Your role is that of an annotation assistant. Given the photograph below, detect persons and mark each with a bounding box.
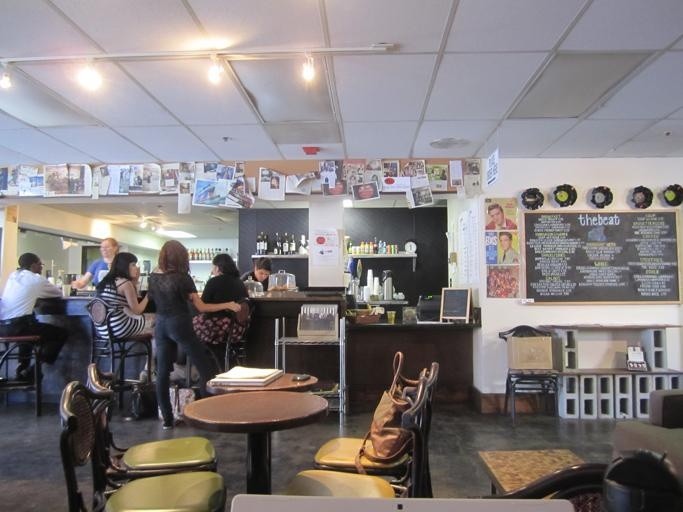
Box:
[93,253,156,380]
[429,169,436,180]
[487,270,518,298]
[240,258,273,291]
[71,238,122,290]
[486,204,517,230]
[440,169,447,180]
[149,241,241,430]
[499,232,519,264]
[170,253,250,381]
[0,253,68,379]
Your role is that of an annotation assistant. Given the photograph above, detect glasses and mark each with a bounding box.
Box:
[34,262,46,268]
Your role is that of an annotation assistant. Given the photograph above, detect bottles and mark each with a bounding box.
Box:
[346,236,398,254]
[255,230,307,255]
[382,270,393,300]
[186,247,229,260]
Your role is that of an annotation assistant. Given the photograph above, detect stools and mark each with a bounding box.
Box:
[647,389,682,428]
[0,335,43,417]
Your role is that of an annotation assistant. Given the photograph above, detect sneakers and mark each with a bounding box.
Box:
[163,421,180,430]
[139,362,199,384]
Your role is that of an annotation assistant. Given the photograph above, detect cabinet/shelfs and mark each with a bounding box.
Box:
[273,317,350,426]
[344,254,417,305]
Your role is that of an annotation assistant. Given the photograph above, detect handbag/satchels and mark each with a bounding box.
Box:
[356,351,431,461]
[158,386,195,422]
[128,389,157,421]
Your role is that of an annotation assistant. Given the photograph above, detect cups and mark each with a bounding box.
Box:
[63,285,71,296]
[363,269,379,301]
[387,312,396,324]
[402,307,417,322]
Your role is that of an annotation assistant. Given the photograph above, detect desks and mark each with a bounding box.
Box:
[477,448,586,500]
[183,390,329,496]
[205,372,321,396]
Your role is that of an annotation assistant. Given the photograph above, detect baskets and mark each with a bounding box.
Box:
[345,307,382,324]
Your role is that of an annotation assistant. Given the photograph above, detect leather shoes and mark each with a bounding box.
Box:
[19,368,44,381]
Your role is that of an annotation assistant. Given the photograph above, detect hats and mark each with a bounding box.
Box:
[18,253,38,270]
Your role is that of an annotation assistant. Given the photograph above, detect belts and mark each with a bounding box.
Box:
[1,313,36,326]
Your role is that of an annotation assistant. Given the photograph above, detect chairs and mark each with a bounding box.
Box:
[84,297,153,412]
[185,296,255,390]
[467,462,610,511]
[283,375,430,498]
[312,361,440,498]
[498,325,561,429]
[85,362,218,490]
[57,380,227,512]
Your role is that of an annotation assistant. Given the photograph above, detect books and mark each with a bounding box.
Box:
[210,362,283,388]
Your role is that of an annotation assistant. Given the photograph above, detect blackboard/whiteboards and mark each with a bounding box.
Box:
[440,287,471,320]
[520,207,683,305]
